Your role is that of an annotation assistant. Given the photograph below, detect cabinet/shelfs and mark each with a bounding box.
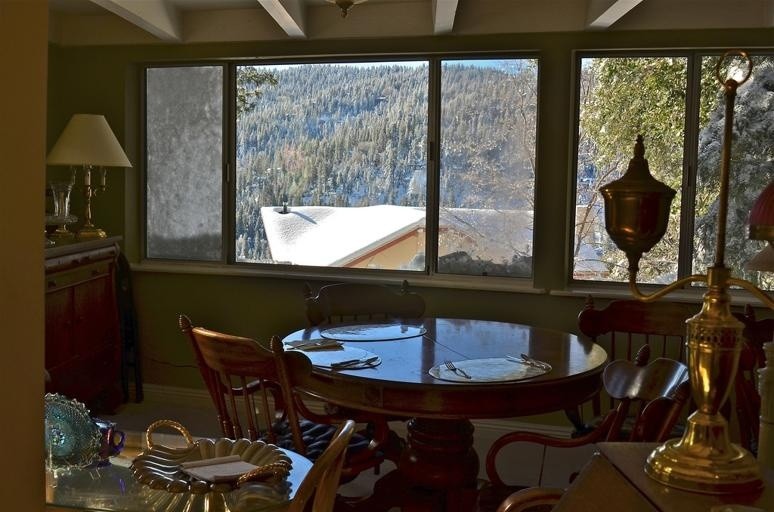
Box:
[45,259,120,407]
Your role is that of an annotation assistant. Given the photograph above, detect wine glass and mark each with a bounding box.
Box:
[50,181,79,237]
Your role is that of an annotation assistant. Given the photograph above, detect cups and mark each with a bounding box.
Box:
[94,418,126,469]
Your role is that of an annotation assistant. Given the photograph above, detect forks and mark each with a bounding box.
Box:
[442,358,475,380]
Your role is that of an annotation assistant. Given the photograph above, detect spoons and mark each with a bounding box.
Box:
[506,351,551,373]
[331,354,380,369]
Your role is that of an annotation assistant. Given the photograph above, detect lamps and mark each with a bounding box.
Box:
[598,46,772,497]
[45,110,134,239]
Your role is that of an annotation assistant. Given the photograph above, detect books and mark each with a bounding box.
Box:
[179,455,263,483]
